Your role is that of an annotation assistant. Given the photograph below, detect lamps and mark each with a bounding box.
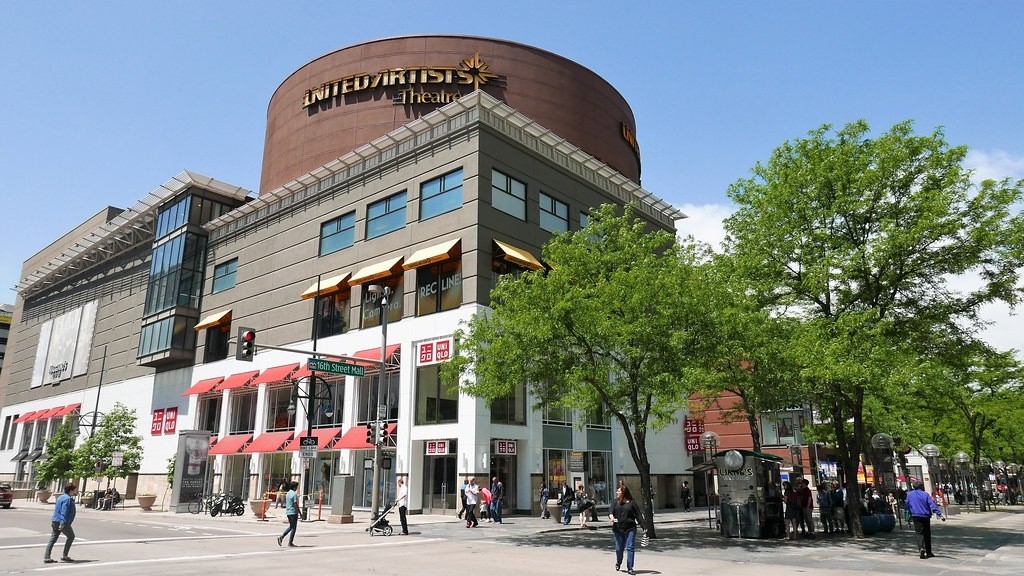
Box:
[77,244,78,247]
[49,263,51,266]
[26,279,28,281]
[109,222,112,225]
[130,210,132,212]
[92,234,94,236]
[62,254,64,256]
[15,286,17,288]
[37,271,39,274]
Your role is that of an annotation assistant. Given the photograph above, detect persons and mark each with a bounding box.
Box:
[457,476,504,528]
[538,482,551,519]
[767,473,845,541]
[277,481,303,547]
[905,480,946,559]
[617,479,625,489]
[857,496,894,517]
[44,482,75,563]
[607,484,648,575]
[274,477,289,510]
[397,478,408,535]
[680,481,691,513]
[557,479,572,524]
[931,482,948,520]
[1017,492,1023,504]
[650,483,656,516]
[95,487,121,511]
[576,484,589,528]
[998,490,1005,500]
[954,489,978,506]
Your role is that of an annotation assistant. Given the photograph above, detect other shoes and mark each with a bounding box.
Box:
[399,533,408,535]
[616,564,620,570]
[97,507,102,510]
[288,542,297,547]
[101,508,107,510]
[564,522,568,525]
[783,536,790,540]
[458,514,461,519]
[277,537,282,545]
[547,516,549,518]
[466,521,472,528]
[920,549,926,559]
[465,515,467,520]
[582,524,587,528]
[44,558,53,563]
[496,521,502,524]
[628,570,636,575]
[473,522,478,527]
[61,556,74,562]
[542,516,545,519]
[926,552,934,558]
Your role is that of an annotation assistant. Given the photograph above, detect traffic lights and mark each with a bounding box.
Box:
[363,458,374,470]
[381,458,392,470]
[236,325,255,362]
[364,424,376,445]
[378,422,389,444]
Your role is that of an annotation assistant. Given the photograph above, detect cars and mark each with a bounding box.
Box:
[0,485,13,508]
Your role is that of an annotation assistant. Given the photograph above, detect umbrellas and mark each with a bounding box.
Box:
[896,474,923,483]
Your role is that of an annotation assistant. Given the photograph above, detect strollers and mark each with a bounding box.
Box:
[366,500,398,536]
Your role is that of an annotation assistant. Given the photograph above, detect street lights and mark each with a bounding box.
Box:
[955,451,972,514]
[287,274,336,525]
[699,431,721,532]
[367,283,394,526]
[920,444,940,507]
[70,344,107,505]
[871,433,894,501]
[995,460,1006,506]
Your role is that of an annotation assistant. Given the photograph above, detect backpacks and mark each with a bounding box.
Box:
[789,491,800,509]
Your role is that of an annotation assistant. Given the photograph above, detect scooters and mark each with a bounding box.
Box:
[210,493,245,518]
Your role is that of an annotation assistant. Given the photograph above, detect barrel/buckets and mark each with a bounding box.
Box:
[873,514,895,531]
[860,515,880,534]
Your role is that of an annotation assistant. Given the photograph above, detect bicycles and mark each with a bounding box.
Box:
[187,492,220,514]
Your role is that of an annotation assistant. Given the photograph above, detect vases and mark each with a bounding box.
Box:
[55,493,63,500]
[873,514,896,533]
[861,515,881,535]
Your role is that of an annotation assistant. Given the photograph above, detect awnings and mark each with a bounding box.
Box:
[11,451,53,462]
[209,424,396,455]
[493,238,544,270]
[13,404,80,423]
[194,309,232,330]
[300,237,461,300]
[181,344,399,396]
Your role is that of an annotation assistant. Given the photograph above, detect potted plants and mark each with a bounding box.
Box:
[137,493,157,510]
[37,489,53,503]
[80,494,94,508]
[249,496,273,517]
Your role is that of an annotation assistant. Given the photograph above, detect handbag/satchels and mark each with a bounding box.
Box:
[641,533,649,547]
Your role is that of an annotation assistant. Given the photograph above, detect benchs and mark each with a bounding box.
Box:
[104,493,127,510]
